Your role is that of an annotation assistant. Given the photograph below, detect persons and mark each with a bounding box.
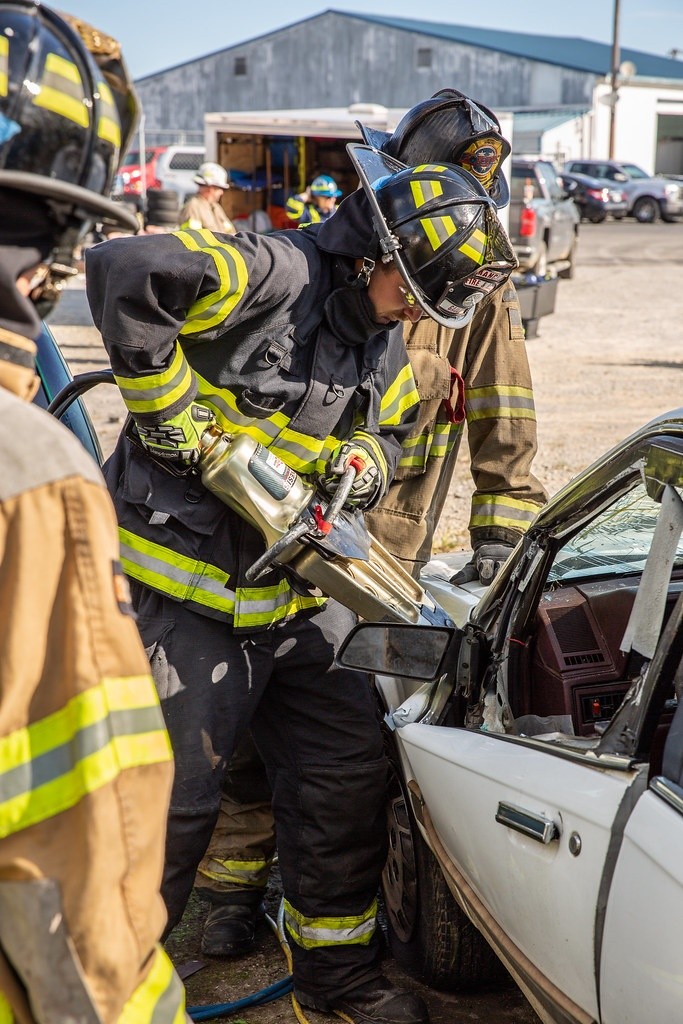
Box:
[284,174,343,230]
[83,161,518,1023]
[0,0,204,1024]
[188,86,552,961]
[178,161,238,238]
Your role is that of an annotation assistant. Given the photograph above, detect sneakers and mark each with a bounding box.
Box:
[200,902,257,956]
[292,978,430,1024]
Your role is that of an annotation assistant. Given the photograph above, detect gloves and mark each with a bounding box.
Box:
[135,403,216,475]
[317,441,381,508]
[449,537,516,585]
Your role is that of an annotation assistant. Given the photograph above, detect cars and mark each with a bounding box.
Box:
[109,146,207,221]
[554,171,628,227]
[333,406,683,1024]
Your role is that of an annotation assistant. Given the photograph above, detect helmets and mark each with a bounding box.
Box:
[1,0,143,262]
[347,140,520,329]
[355,88,510,210]
[310,175,342,198]
[194,161,229,189]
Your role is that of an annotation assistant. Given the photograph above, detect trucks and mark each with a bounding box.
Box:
[200,103,512,322]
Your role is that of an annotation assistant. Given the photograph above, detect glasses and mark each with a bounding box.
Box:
[387,264,425,313]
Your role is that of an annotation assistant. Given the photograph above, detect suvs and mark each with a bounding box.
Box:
[566,159,683,225]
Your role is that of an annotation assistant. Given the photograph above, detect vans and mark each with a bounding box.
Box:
[509,157,586,282]
